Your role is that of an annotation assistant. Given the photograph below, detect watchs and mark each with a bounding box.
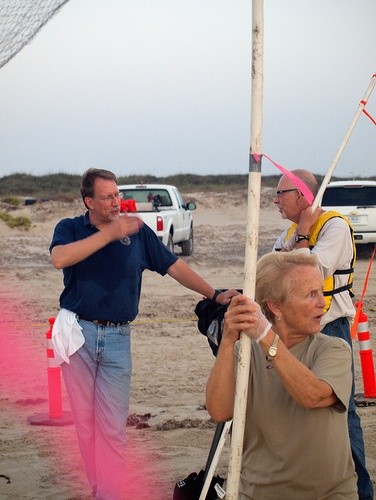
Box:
[294,233,310,243]
[266,333,279,360]
[212,289,221,302]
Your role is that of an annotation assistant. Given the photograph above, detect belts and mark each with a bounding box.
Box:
[91,318,131,328]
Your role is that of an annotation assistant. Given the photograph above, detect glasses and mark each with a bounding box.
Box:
[276,189,303,198]
[90,191,123,203]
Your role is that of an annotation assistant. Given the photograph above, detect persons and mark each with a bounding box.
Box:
[206,252,359,500]
[49,167,242,500]
[272,169,374,500]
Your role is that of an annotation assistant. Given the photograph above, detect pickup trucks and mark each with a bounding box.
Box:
[116,183,196,257]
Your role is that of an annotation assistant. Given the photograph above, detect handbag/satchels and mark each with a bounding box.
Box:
[174,468,224,500]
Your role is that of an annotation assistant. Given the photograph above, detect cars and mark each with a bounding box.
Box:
[318,180,376,244]
